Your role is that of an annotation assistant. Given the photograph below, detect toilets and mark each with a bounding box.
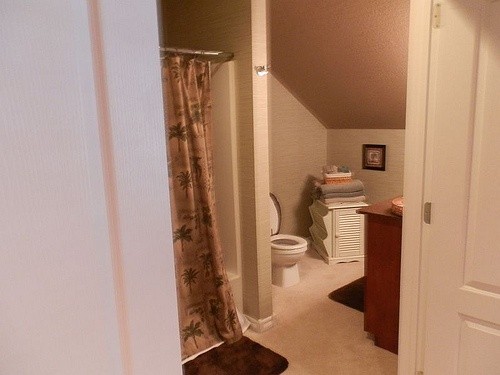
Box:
[270,193,308,288]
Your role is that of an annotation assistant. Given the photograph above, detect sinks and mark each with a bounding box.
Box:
[392,196,404,216]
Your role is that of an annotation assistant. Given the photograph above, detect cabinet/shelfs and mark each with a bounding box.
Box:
[356,193,404,355]
[309,199,370,265]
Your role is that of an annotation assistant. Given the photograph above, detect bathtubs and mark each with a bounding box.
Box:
[226,271,243,314]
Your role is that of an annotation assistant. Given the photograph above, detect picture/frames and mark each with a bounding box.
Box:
[362,144,386,171]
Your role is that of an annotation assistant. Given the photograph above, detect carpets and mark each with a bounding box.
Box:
[182,336,289,375]
[328,276,364,312]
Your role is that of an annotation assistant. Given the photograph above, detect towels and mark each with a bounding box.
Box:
[311,180,367,205]
